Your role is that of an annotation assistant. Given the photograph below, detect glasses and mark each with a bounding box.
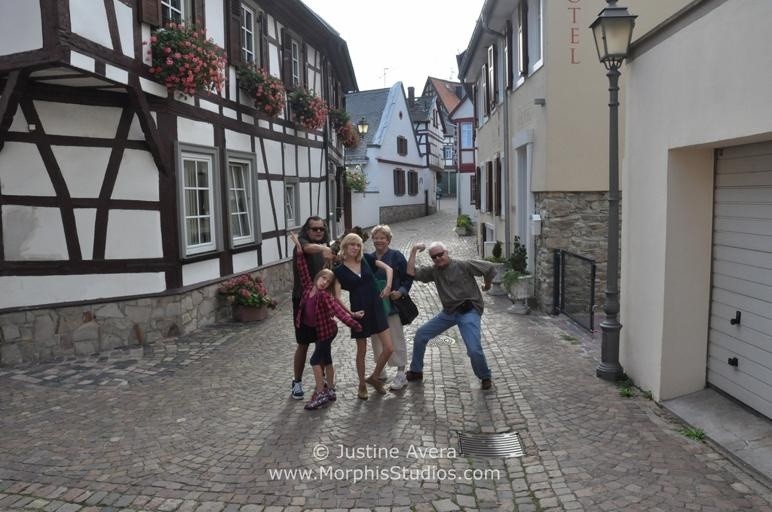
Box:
[431,252,444,259]
[310,227,325,231]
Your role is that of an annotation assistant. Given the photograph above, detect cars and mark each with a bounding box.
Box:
[436,186,443,199]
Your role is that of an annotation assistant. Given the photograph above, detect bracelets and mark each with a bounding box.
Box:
[352,312,355,317]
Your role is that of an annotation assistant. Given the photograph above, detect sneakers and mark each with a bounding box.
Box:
[482,379,491,389]
[290,374,337,409]
[358,369,423,399]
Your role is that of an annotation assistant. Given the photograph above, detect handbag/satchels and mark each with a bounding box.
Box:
[393,294,418,324]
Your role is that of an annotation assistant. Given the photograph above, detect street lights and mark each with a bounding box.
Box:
[590,0,638,381]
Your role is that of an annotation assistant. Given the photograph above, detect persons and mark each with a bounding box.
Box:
[368,223,415,391]
[287,230,364,411]
[333,233,395,400]
[289,215,336,401]
[404,241,498,390]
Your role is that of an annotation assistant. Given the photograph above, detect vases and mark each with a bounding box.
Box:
[231,303,268,322]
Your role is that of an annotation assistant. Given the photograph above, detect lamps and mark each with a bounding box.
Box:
[357,116,369,139]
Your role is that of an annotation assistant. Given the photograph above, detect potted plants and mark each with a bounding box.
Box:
[452,214,473,236]
[480,241,508,296]
[500,234,536,315]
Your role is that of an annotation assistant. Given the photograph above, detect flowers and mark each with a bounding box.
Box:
[217,272,278,311]
[142,13,359,149]
